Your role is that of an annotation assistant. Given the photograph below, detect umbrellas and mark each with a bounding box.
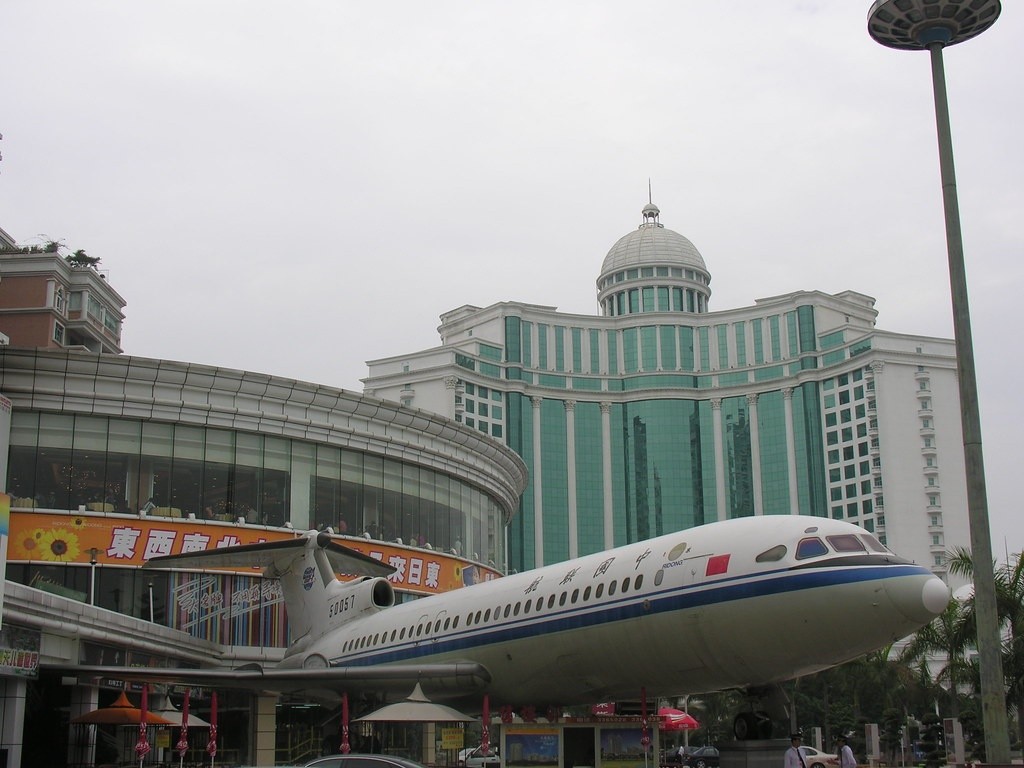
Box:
[640,689,650,768]
[482,696,489,768]
[659,708,698,762]
[207,692,218,768]
[70,690,174,724]
[349,681,479,721]
[177,687,190,768]
[136,684,150,768]
[150,696,213,727]
[340,690,350,755]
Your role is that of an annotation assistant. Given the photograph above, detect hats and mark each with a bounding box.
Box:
[788,733,802,739]
[837,734,849,741]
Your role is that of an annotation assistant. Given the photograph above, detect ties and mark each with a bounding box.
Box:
[840,749,842,768]
[797,748,806,768]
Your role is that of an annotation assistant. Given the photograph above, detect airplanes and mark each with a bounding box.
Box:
[0,513,952,741]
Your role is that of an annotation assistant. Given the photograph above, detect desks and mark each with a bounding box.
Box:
[214,514,235,521]
[86,502,114,512]
[13,498,38,508]
[150,507,181,518]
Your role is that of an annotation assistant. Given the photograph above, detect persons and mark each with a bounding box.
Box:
[677,745,685,762]
[784,736,807,768]
[144,498,156,515]
[206,505,215,520]
[829,736,857,768]
[336,515,347,534]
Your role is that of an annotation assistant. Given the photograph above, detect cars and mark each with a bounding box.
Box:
[301,753,427,768]
[799,746,840,768]
[459,748,477,767]
[465,743,500,768]
[661,747,700,763]
[683,746,720,768]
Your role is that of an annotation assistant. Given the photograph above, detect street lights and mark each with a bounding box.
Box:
[867,0,1016,768]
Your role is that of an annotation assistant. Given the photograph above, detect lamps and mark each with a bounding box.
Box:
[78,506,518,574]
